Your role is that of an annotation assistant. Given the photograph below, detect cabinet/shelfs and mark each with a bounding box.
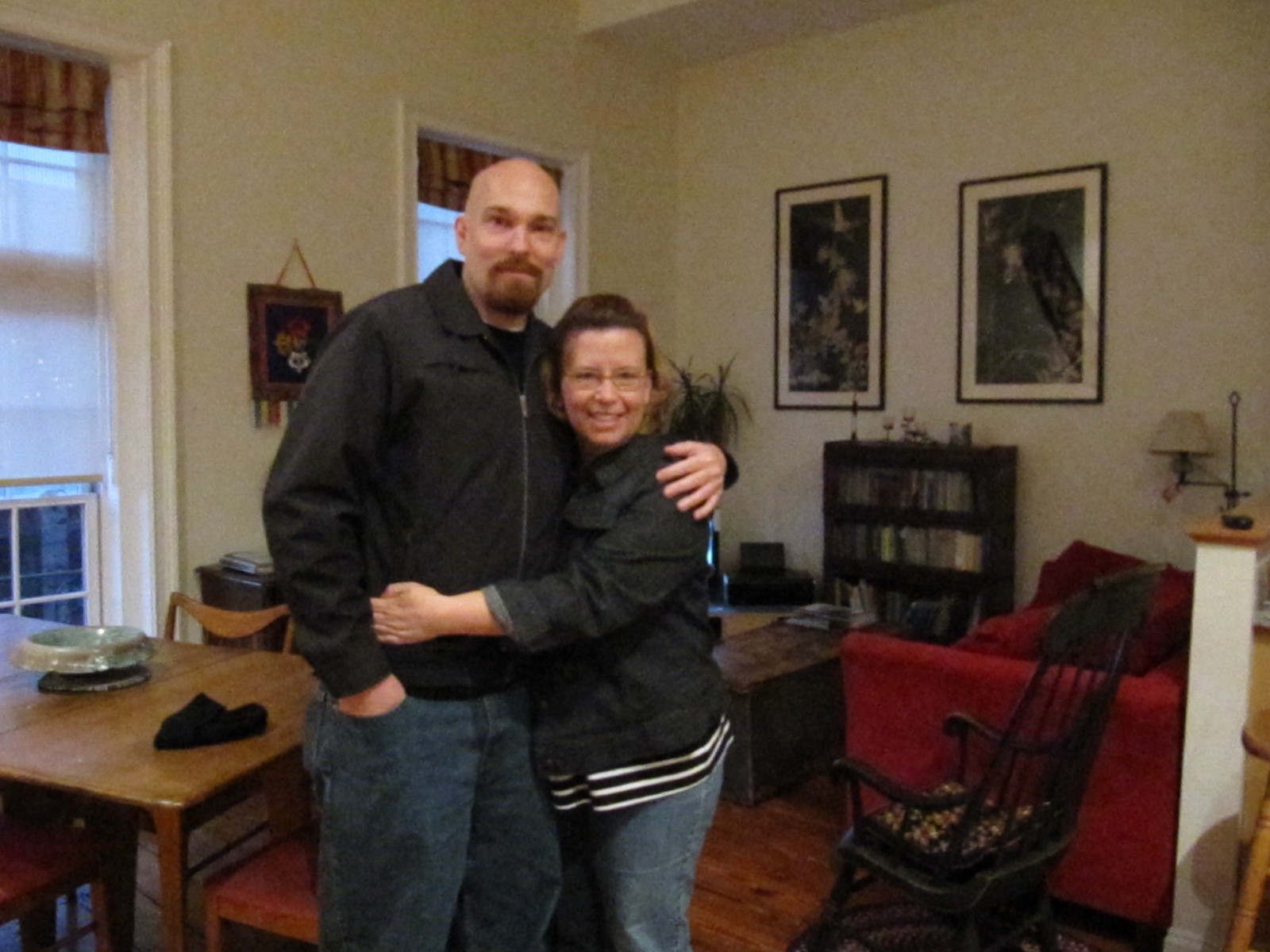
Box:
[824,440,1024,632]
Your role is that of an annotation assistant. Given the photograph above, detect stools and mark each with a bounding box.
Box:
[1225,711,1270,952]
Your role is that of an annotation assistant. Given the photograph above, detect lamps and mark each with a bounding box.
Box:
[1151,413,1247,514]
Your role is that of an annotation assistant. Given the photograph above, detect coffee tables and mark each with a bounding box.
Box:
[711,620,897,805]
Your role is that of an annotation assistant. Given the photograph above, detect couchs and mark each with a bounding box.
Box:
[849,542,1202,926]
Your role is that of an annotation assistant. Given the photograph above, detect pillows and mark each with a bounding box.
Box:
[952,601,1057,654]
[1075,573,1195,671]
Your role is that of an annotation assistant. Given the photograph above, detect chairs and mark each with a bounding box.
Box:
[821,563,1170,952]
[0,590,319,952]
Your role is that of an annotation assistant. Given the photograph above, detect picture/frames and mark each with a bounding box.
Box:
[247,284,348,404]
[954,162,1108,406]
[770,173,886,411]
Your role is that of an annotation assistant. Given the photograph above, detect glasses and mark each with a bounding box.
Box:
[560,367,654,392]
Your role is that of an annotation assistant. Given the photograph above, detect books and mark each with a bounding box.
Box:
[834,467,984,641]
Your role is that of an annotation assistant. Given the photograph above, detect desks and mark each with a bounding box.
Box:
[0,613,322,952]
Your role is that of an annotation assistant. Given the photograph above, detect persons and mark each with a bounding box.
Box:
[371,294,734,952]
[259,155,741,951]
